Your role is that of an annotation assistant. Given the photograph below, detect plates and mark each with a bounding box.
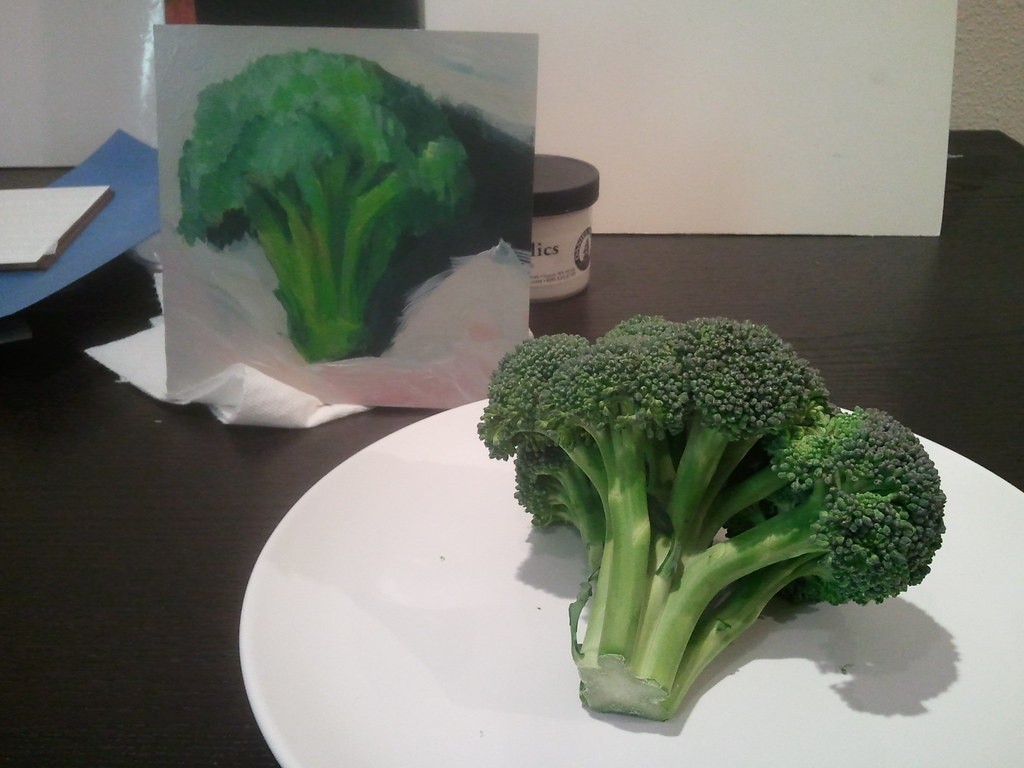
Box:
[236,397,1024,768]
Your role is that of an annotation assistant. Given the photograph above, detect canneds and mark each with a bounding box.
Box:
[531,155,599,302]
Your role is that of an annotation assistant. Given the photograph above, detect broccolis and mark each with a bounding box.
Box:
[476,315,947,720]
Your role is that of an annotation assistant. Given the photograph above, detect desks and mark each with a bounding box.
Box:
[0,128,1024,768]
